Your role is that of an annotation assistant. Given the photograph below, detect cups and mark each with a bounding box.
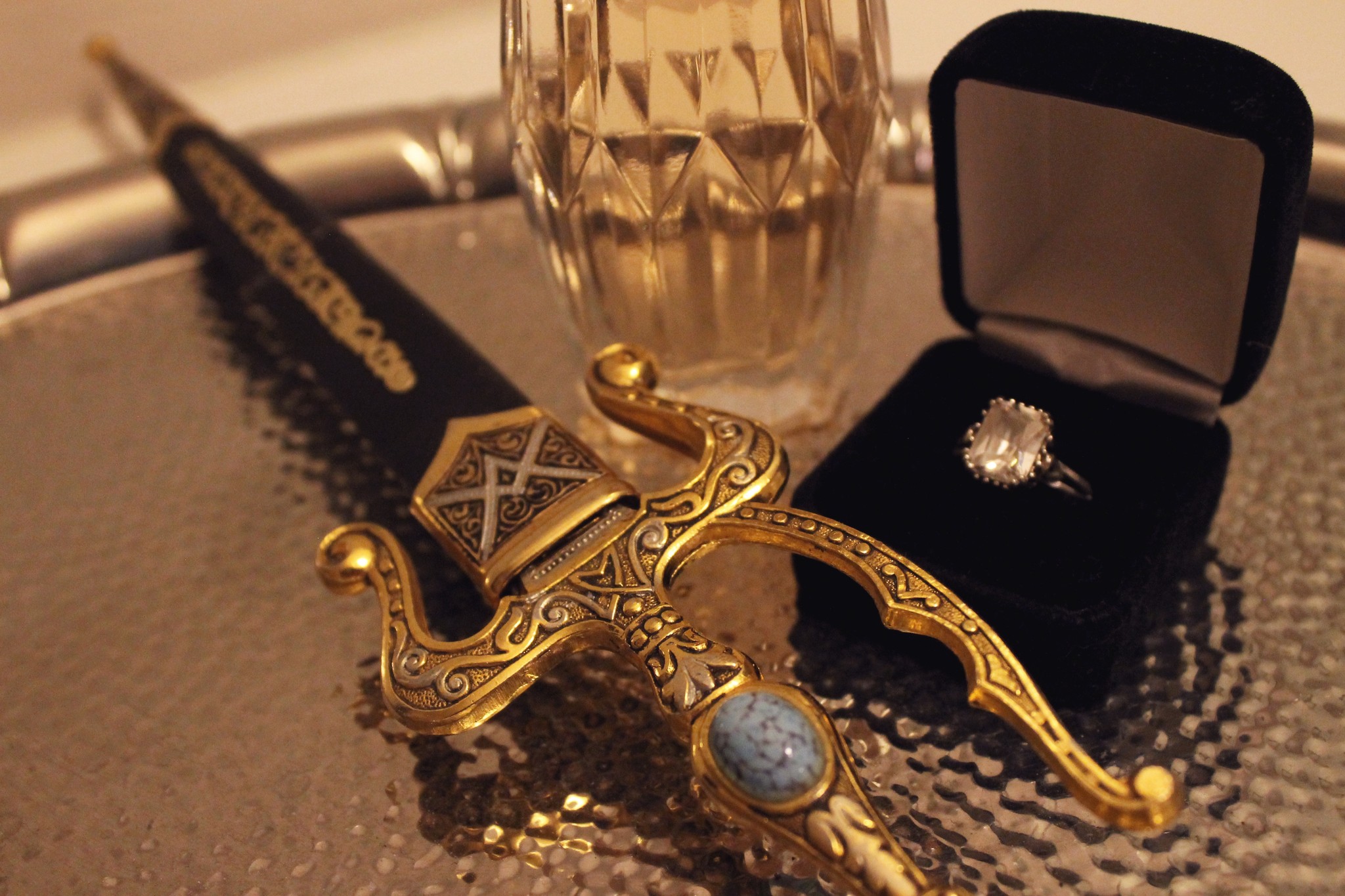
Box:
[500,0,896,442]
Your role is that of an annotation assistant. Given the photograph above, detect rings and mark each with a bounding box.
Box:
[961,398,1094,506]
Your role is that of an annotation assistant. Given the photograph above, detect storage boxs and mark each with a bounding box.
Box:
[784,11,1315,706]
